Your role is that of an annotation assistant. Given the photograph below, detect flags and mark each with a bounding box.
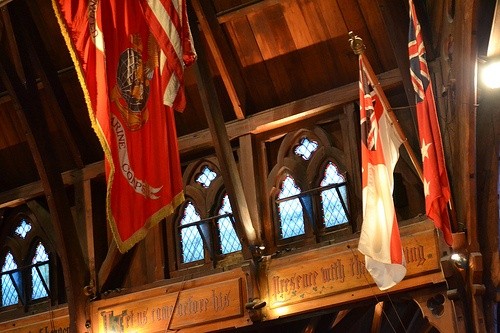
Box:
[139,0,199,115]
[408,5,458,246]
[355,53,405,293]
[54,1,186,253]
